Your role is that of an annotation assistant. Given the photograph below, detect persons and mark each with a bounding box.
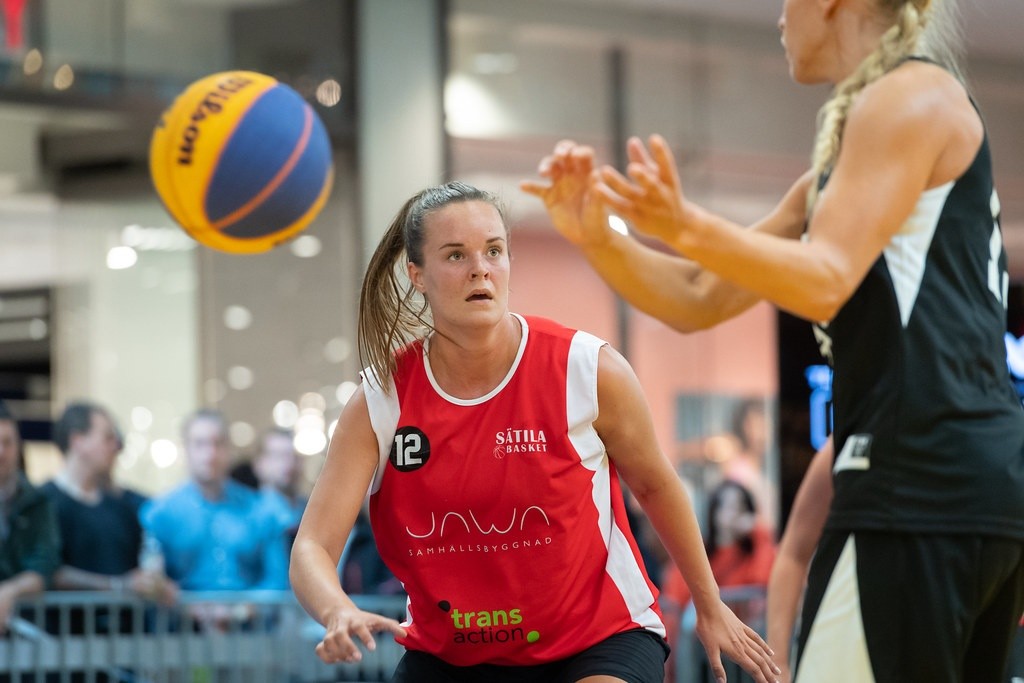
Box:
[516,0,1024,683]
[0,399,835,683]
[287,182,781,683]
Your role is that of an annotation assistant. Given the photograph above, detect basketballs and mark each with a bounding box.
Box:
[144,66,339,258]
